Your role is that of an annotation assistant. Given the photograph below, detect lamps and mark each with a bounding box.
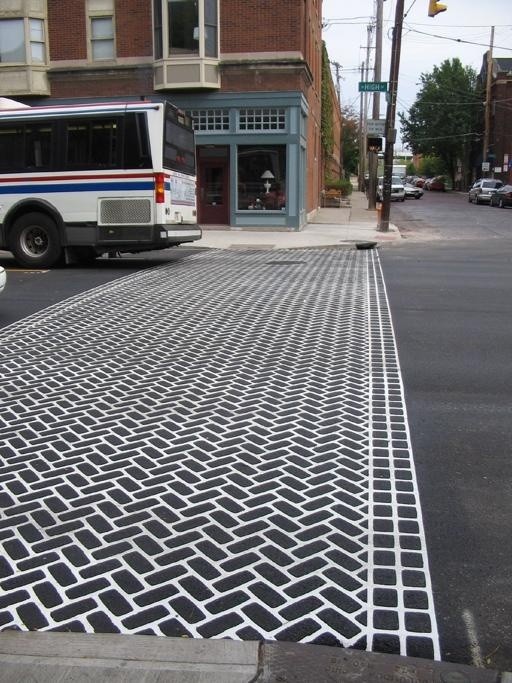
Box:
[260,169,275,193]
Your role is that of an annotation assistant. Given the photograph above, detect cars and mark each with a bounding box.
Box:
[364,172,453,201]
[489,183,512,208]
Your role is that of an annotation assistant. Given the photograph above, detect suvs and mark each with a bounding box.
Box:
[468,176,505,205]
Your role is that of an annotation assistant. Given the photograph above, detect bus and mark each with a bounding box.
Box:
[1,96,205,269]
